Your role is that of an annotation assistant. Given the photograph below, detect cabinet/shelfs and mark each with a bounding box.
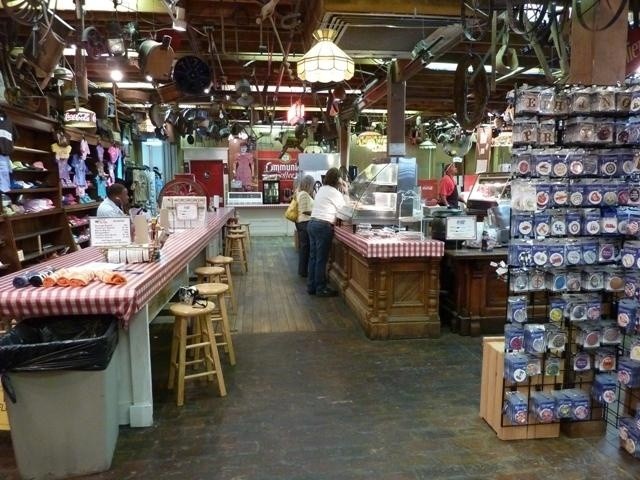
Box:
[0,100,130,336]
[336,156,416,225]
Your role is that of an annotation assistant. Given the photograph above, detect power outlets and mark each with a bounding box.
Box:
[159,0,188,32]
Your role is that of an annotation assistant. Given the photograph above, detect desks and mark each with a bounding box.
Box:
[326,224,617,341]
[0,204,237,429]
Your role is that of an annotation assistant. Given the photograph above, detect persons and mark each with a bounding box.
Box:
[292,174,315,278]
[435,162,468,210]
[96,182,139,238]
[232,141,256,192]
[306,167,349,298]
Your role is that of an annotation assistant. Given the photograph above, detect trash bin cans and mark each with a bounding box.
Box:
[0,315,118,480]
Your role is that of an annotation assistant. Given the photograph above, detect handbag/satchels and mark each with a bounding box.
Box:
[284,198,298,221]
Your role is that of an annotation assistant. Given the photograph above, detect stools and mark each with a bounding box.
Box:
[166,216,252,407]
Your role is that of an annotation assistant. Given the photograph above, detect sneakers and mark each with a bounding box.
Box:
[307,286,338,297]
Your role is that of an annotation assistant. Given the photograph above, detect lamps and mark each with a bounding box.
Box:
[419,137,437,150]
[356,131,383,148]
[296,11,355,85]
